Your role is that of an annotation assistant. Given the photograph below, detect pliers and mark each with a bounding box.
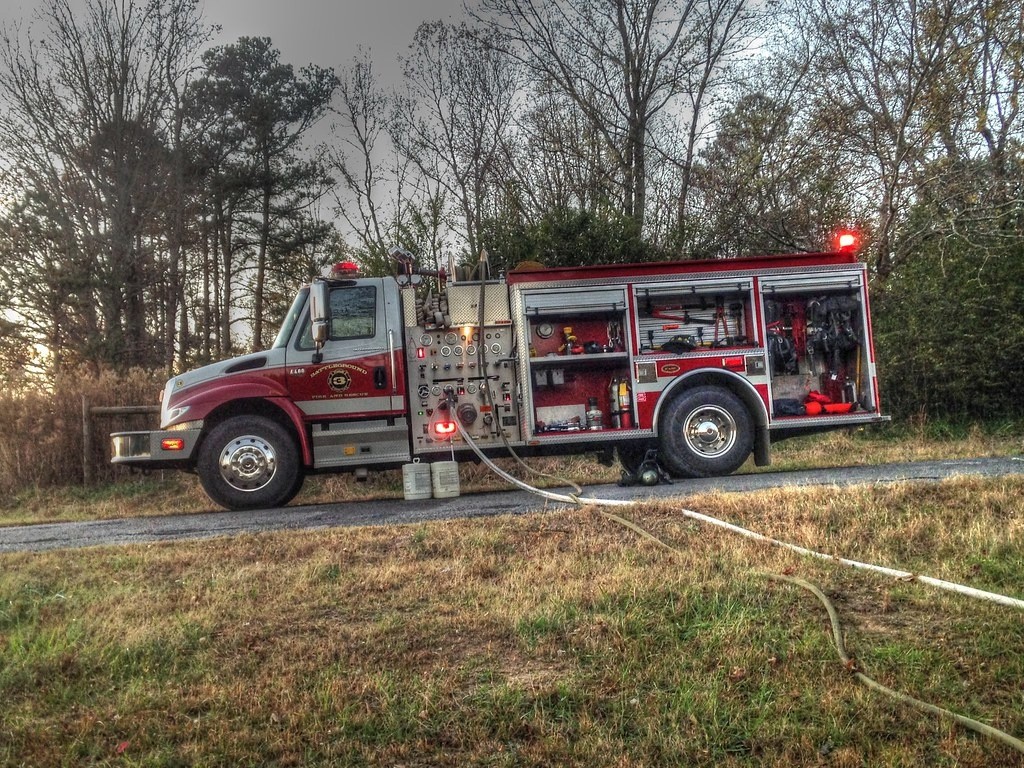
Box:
[636,303,716,324]
[714,307,729,346]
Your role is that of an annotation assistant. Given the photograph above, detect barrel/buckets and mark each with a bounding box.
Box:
[401,462,431,500]
[430,460,460,499]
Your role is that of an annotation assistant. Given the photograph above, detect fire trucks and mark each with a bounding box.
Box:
[110,226,893,512]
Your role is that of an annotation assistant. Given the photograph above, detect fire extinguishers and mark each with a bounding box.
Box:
[608,375,620,429]
[618,376,633,429]
